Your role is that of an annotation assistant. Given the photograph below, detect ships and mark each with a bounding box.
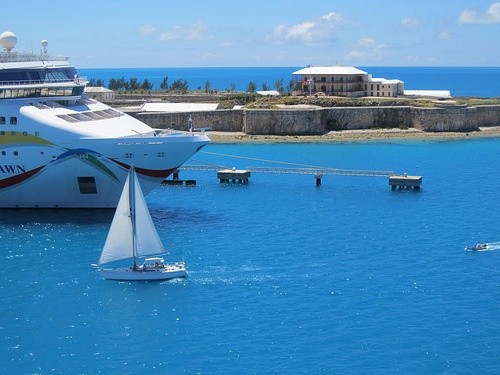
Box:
[1,32,211,211]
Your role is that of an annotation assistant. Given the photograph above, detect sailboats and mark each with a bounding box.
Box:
[89,162,190,284]
